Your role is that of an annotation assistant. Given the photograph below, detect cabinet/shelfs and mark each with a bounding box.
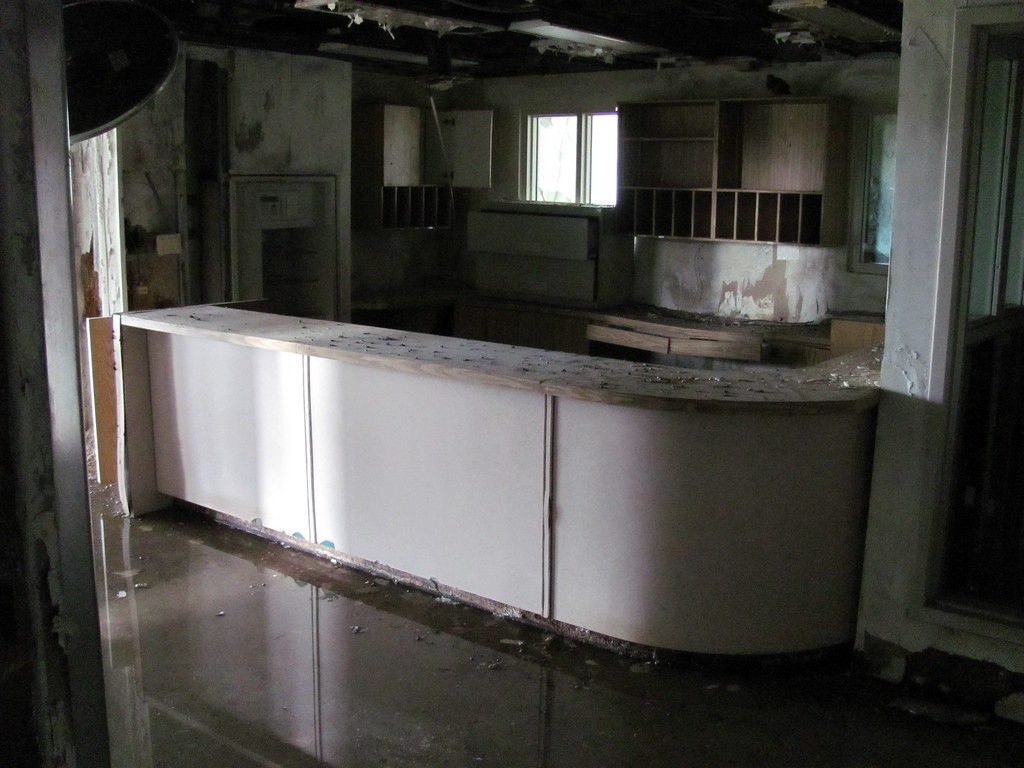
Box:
[368,100,496,232]
[610,94,852,249]
